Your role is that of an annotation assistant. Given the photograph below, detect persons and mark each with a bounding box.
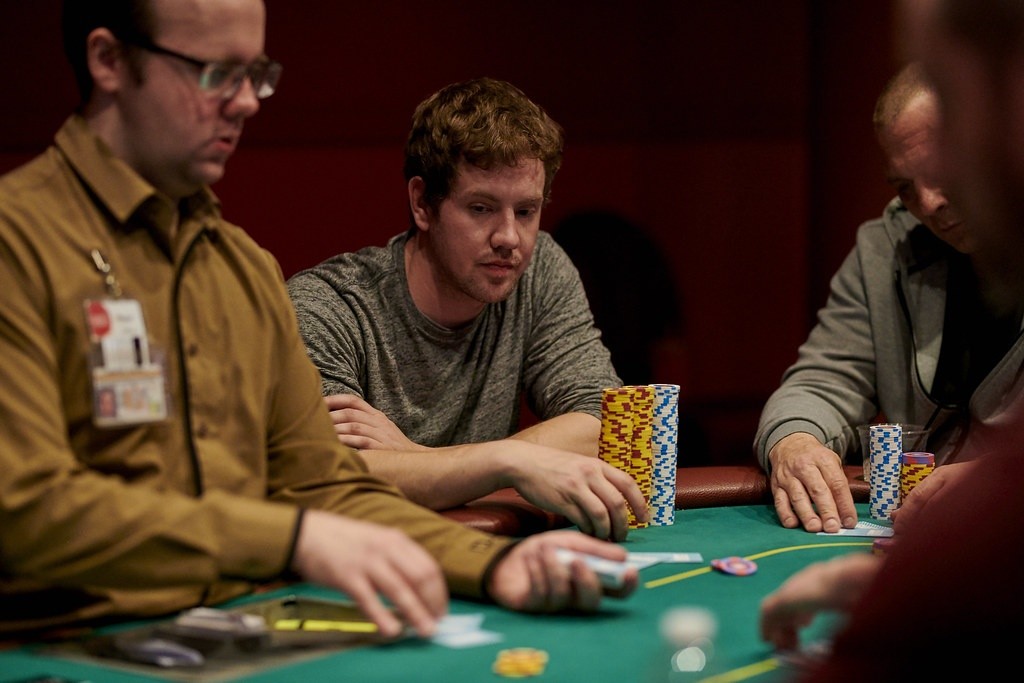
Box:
[752,57,1024,534]
[288,74,649,543]
[756,2,1023,682]
[0,0,640,641]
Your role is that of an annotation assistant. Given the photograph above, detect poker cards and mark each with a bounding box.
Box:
[816,520,895,538]
[554,548,704,591]
[403,612,505,651]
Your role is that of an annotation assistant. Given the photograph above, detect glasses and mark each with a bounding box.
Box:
[118,33,281,99]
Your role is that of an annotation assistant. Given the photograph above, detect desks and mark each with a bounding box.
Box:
[1,466,937,683]
